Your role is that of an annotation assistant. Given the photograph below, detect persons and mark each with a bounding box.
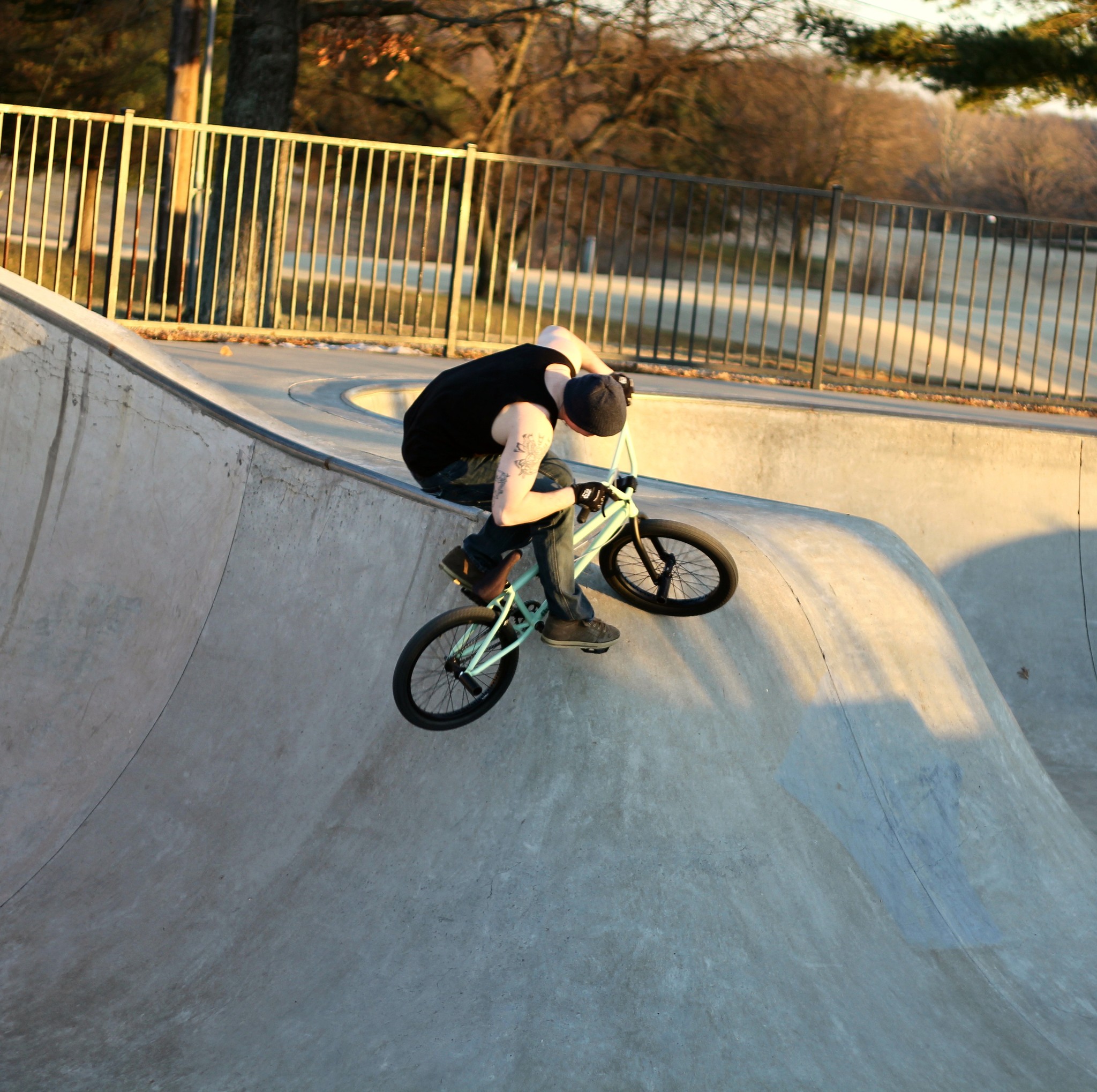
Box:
[401,325,633,648]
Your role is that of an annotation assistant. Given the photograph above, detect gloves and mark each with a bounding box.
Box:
[610,373,634,407]
[572,482,609,512]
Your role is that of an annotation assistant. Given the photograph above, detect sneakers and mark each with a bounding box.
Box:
[439,546,508,590]
[541,616,621,649]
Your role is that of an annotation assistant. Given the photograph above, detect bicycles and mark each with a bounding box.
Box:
[391,405,740,732]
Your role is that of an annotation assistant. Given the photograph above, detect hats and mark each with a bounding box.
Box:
[563,374,627,437]
